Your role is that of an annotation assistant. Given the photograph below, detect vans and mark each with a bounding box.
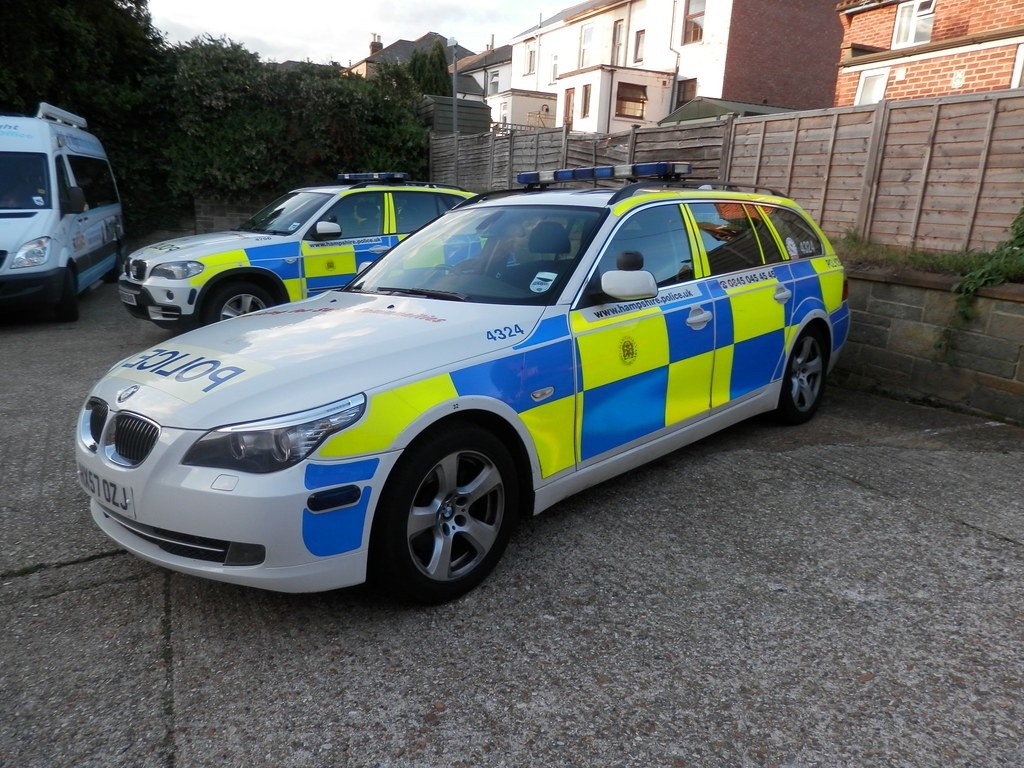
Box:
[0,100,126,324]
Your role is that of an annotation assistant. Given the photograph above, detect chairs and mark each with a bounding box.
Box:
[515,220,572,299]
[357,202,380,229]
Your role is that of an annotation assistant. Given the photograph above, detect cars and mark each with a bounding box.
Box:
[115,171,532,334]
[70,158,851,611]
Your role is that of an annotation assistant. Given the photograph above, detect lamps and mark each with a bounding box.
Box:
[542,104,549,112]
